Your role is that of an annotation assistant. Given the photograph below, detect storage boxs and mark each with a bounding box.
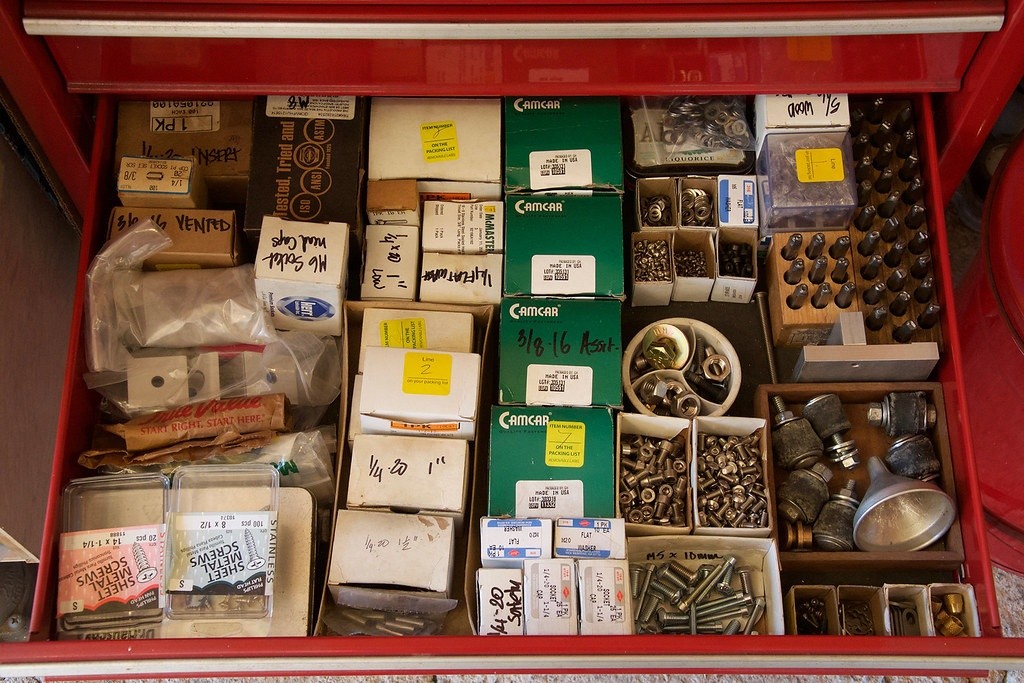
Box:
[62,93,981,637]
